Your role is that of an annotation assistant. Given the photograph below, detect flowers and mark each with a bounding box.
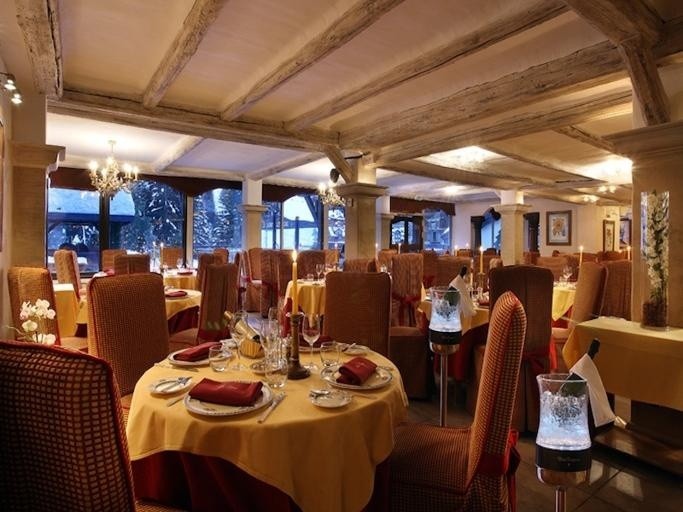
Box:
[643,189,669,330]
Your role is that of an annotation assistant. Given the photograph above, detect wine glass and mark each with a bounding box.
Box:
[176,257,183,272]
[302,311,320,374]
[231,312,250,372]
[316,263,324,283]
[259,319,282,382]
[268,307,283,327]
[563,266,570,287]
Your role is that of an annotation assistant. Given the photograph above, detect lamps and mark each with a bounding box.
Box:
[88,140,140,201]
[0,72,23,107]
[318,178,348,207]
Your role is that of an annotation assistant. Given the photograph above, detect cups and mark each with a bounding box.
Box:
[264,359,289,388]
[207,346,232,371]
[306,274,314,281]
[320,341,341,366]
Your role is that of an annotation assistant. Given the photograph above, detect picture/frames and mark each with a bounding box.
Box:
[619,218,631,249]
[546,209,572,245]
[603,220,616,253]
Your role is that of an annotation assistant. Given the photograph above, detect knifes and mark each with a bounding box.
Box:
[259,391,286,423]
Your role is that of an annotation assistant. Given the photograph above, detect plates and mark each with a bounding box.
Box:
[310,389,351,409]
[184,378,274,417]
[320,363,390,389]
[169,343,230,368]
[151,375,192,396]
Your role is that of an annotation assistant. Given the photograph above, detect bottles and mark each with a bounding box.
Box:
[223,309,261,342]
[434,267,469,320]
[553,340,600,428]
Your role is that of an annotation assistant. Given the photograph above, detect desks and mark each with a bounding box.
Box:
[562,315,682,485]
